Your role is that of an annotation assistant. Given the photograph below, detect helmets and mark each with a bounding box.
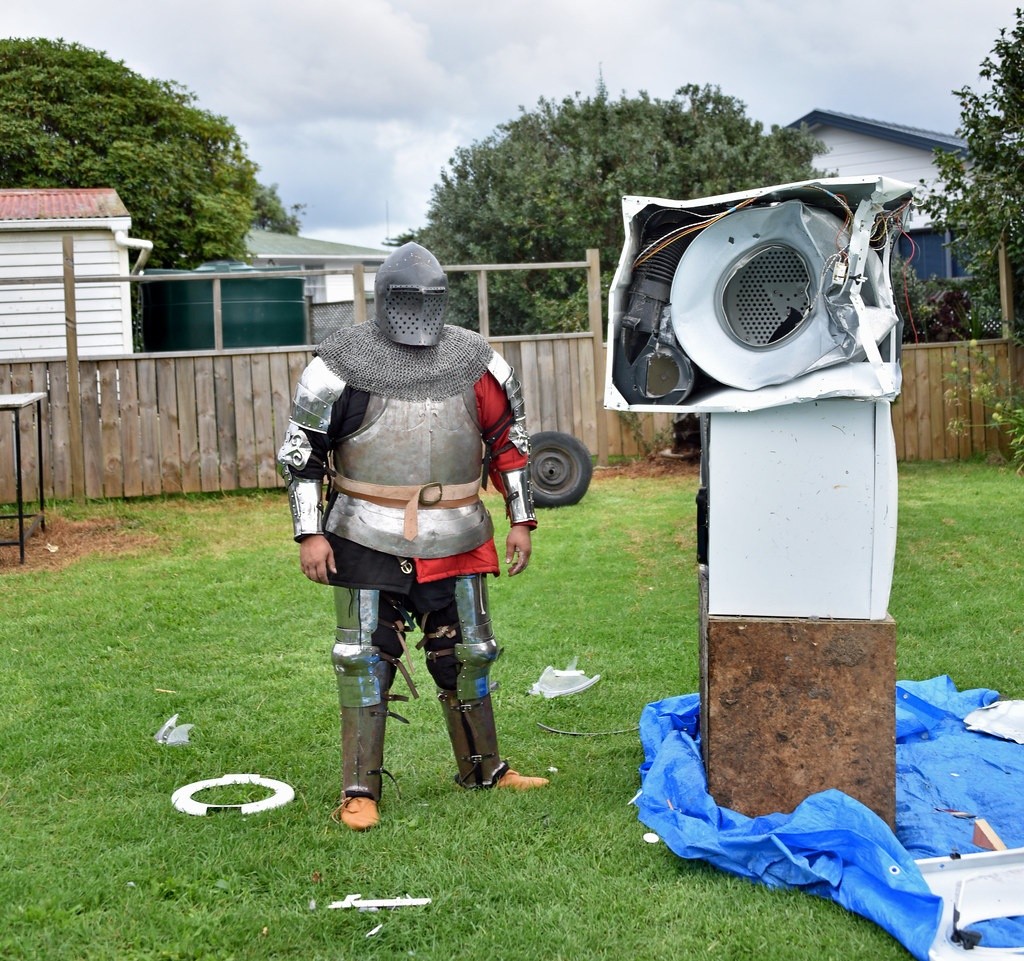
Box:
[374,242,449,345]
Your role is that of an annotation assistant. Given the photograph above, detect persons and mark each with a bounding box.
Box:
[275,239,550,829]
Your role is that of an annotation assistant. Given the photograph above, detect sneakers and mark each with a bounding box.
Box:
[332,797,380,831]
[495,768,549,790]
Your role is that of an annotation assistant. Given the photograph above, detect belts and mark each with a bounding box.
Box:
[331,467,481,542]
